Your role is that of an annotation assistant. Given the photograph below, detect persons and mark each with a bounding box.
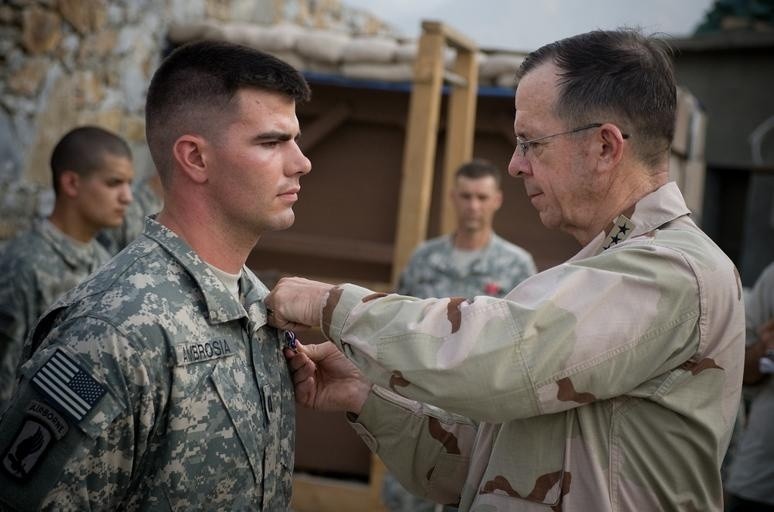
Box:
[264,29,744,512]
[382,161,536,512]
[0,126,139,400]
[0,37,316,512]
[724,261,774,512]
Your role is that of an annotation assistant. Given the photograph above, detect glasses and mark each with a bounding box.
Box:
[517,123,629,158]
